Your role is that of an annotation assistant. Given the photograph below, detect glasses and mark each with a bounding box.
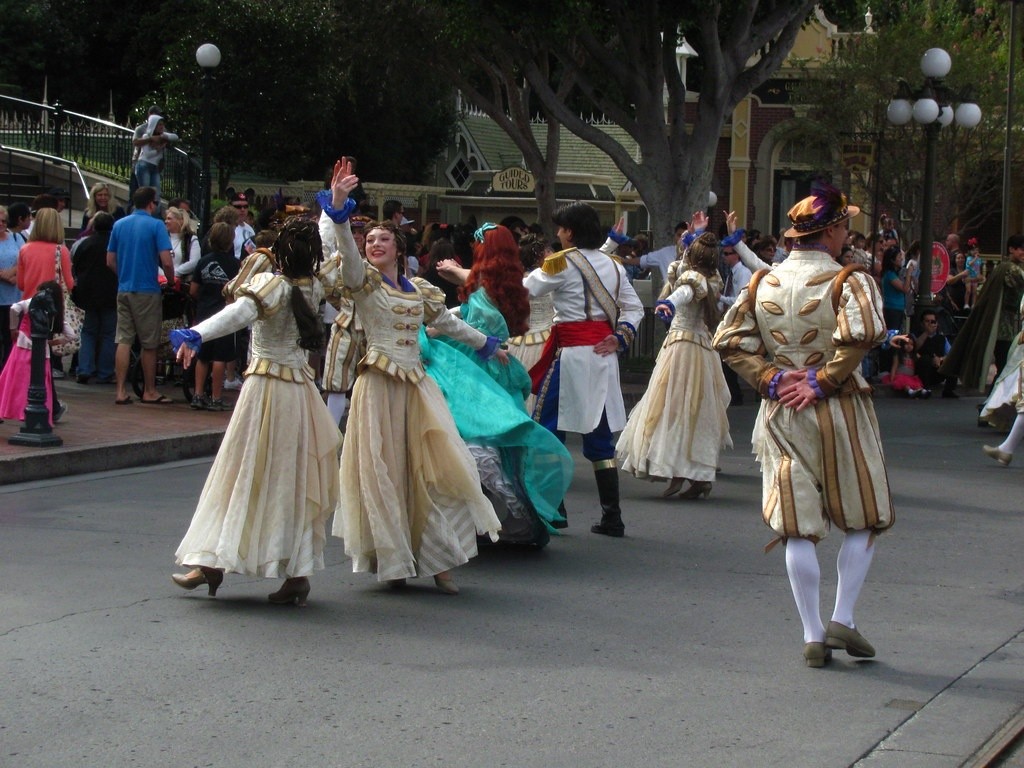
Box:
[397,212,405,215]
[232,205,248,209]
[723,252,737,256]
[524,230,528,235]
[764,248,777,253]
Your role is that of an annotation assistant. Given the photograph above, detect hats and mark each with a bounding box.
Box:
[230,192,247,201]
[350,216,373,227]
[48,188,70,200]
[783,179,860,237]
[399,216,415,225]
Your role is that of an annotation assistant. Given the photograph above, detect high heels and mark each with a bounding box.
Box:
[664,476,684,496]
[269,576,311,607]
[682,481,712,500]
[172,566,223,597]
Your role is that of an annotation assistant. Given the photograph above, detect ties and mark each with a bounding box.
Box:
[723,269,733,311]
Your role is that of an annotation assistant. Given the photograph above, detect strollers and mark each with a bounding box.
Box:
[130,281,211,399]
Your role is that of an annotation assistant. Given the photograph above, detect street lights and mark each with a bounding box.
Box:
[707,192,718,232]
[196,43,223,235]
[886,46,983,338]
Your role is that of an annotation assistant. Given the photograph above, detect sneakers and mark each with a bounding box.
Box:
[52,403,67,422]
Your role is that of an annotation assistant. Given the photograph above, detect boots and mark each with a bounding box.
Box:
[532,453,567,528]
[591,458,624,537]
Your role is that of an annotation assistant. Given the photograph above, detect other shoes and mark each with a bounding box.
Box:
[942,391,959,398]
[983,445,1013,467]
[52,366,243,410]
[910,389,921,398]
[922,390,931,399]
[804,641,831,668]
[434,572,460,593]
[964,304,970,312]
[825,621,876,657]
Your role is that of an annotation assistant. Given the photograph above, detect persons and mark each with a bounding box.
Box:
[134,116,179,214]
[168,217,341,606]
[127,105,172,214]
[0,157,1024,594]
[710,184,893,667]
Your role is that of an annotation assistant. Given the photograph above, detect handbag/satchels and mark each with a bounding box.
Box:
[48,245,85,356]
[159,314,188,361]
